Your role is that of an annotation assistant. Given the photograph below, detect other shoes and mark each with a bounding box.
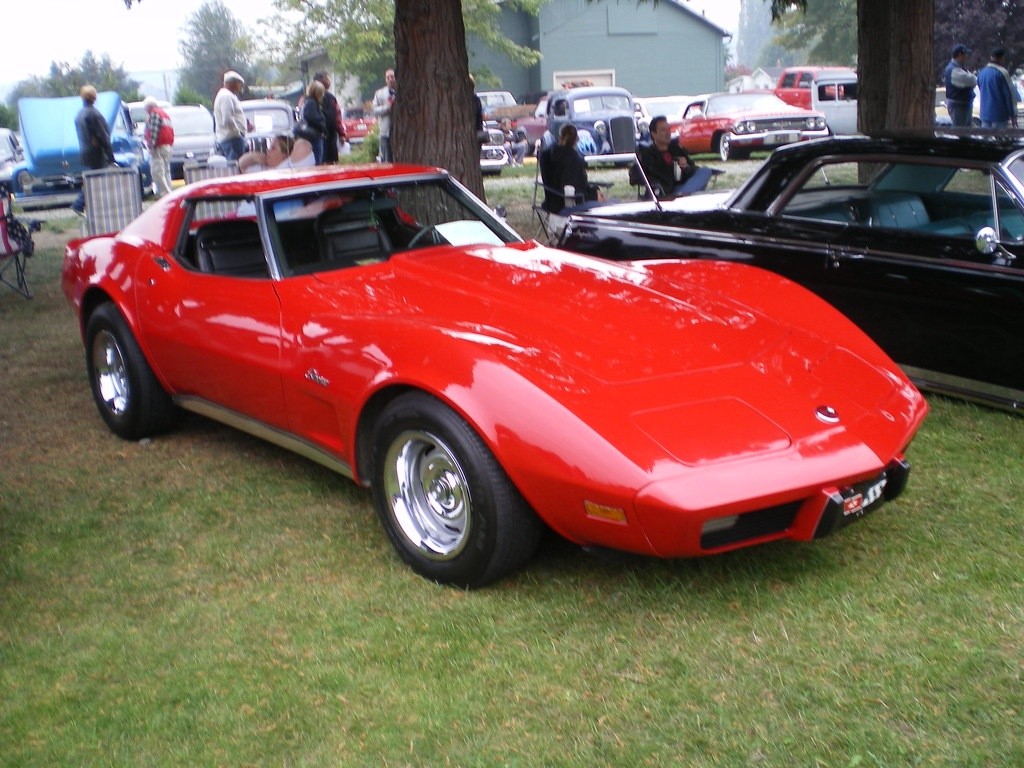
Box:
[71,206,87,218]
[516,161,524,168]
[510,161,515,169]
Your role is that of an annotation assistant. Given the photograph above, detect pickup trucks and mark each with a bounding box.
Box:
[742,67,852,109]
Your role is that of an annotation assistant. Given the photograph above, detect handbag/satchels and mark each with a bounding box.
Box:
[293,120,319,143]
[5,215,34,258]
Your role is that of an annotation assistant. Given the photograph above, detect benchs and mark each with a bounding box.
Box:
[803,190,929,229]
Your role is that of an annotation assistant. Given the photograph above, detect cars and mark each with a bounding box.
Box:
[478,91,519,172]
[10,93,152,211]
[667,89,831,160]
[555,139,1024,420]
[511,85,653,169]
[125,102,216,179]
[937,86,1024,135]
[0,129,23,195]
[809,74,858,137]
[342,108,376,143]
[238,98,299,154]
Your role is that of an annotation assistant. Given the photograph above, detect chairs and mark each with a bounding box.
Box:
[917,210,1024,241]
[184,159,242,186]
[81,167,144,238]
[0,183,40,299]
[629,162,726,203]
[534,180,614,247]
[193,218,268,281]
[315,207,396,275]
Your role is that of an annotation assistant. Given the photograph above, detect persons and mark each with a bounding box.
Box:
[975,47,1017,127]
[236,70,349,176]
[468,74,549,166]
[70,86,114,217]
[943,42,979,140]
[373,69,396,164]
[540,123,610,206]
[628,117,712,201]
[213,71,246,166]
[142,97,174,201]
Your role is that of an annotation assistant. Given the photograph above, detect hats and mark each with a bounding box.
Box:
[990,47,1006,58]
[951,44,972,58]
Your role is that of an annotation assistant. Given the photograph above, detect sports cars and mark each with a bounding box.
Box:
[60,163,933,588]
